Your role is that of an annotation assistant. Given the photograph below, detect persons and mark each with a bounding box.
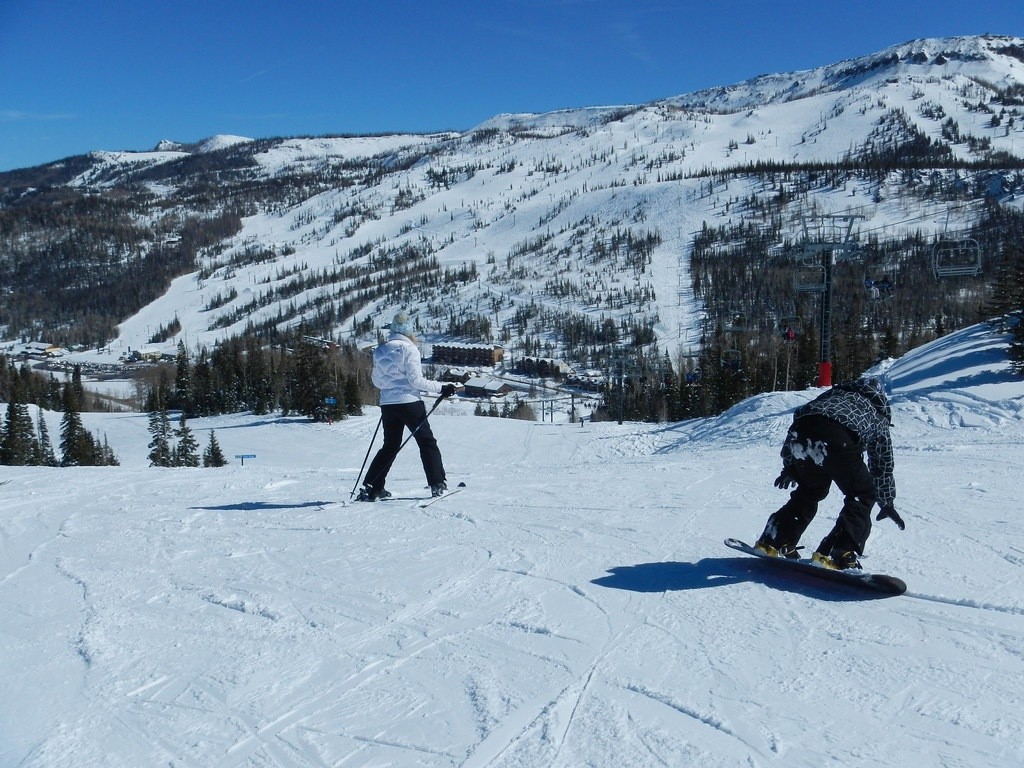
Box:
[360,312,456,501]
[567,406,576,415]
[579,417,584,428]
[583,402,596,409]
[754,378,905,571]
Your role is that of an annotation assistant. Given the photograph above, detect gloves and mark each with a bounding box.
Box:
[876,505,905,530]
[774,469,796,489]
[441,384,458,398]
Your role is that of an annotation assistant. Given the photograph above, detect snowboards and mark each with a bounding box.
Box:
[723,534,909,598]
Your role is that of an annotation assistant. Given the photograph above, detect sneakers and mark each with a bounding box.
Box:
[755,532,805,561]
[365,486,391,499]
[812,540,863,573]
[431,481,449,497]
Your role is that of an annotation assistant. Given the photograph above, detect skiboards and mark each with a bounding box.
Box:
[316,480,469,510]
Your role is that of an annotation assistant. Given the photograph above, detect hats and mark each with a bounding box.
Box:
[390,312,416,337]
